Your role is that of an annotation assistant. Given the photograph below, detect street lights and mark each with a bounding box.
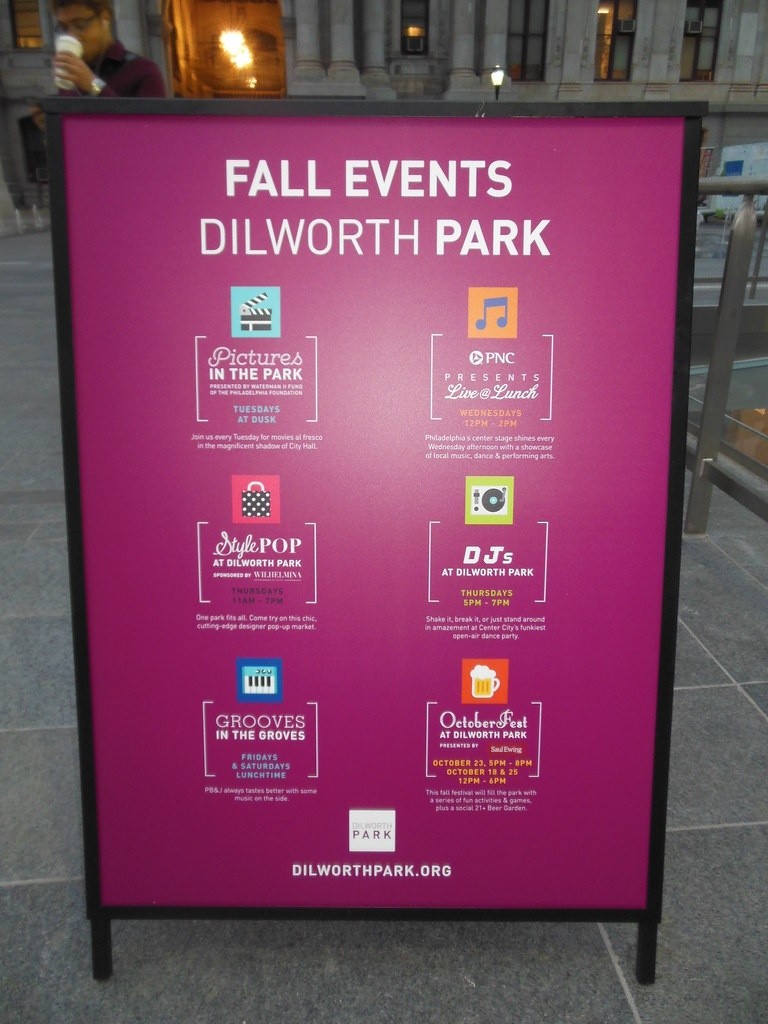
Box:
[490,63,504,100]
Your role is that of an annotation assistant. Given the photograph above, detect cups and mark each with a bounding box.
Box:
[54,36,84,90]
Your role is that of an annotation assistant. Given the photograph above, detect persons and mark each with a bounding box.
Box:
[29,0,165,133]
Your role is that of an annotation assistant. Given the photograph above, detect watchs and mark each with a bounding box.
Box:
[89,76,106,97]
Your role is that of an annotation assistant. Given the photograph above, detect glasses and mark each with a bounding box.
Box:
[53,12,100,34]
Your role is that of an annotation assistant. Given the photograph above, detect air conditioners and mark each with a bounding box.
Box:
[401,37,424,52]
[684,19,703,34]
[617,18,636,33]
